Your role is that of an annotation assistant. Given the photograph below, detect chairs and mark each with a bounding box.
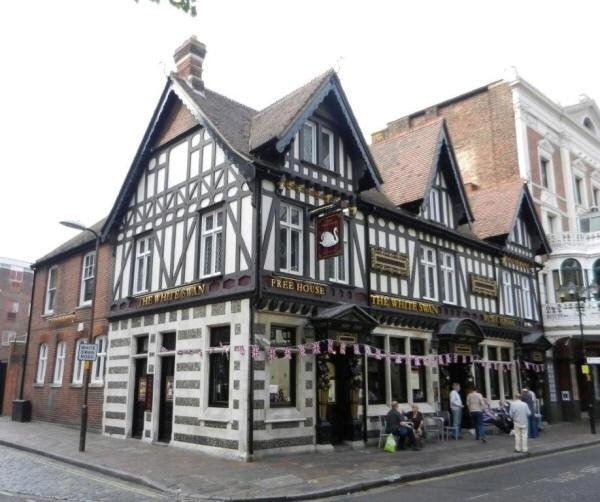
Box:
[378,410,459,450]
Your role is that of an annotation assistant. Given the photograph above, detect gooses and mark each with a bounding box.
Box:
[319,225,340,249]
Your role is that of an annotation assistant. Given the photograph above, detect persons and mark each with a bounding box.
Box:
[449,382,545,453]
[385,400,427,452]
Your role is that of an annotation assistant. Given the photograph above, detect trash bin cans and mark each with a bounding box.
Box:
[12,399,32,422]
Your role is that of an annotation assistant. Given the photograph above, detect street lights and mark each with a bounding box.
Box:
[59,221,99,451]
[559,283,599,435]
